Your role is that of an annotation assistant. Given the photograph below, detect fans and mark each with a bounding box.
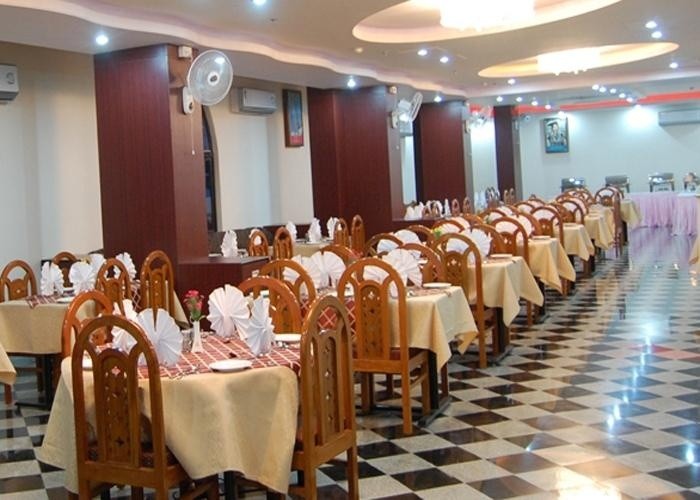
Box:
[180,49,235,114]
[465,105,494,133]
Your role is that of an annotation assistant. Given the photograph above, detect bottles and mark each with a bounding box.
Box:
[181,330,194,353]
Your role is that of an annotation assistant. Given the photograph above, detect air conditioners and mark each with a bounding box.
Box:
[228,86,277,117]
[0,60,22,101]
[658,107,700,128]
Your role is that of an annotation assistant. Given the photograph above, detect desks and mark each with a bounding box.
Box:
[264,276,481,428]
[0,280,189,394]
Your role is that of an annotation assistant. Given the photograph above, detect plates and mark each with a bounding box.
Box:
[210,359,253,372]
[423,282,451,289]
[490,254,514,259]
[208,253,222,257]
[57,297,77,303]
[323,292,355,297]
[295,234,334,245]
[532,235,551,239]
[56,287,74,292]
[272,332,303,342]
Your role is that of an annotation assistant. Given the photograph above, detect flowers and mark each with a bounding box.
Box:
[389,89,424,132]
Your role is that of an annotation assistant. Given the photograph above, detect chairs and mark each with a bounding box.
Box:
[222,294,361,500]
[429,233,502,367]
[94,257,133,316]
[338,258,435,435]
[138,249,175,316]
[239,276,304,339]
[51,251,79,295]
[58,290,117,356]
[178,185,630,325]
[71,313,221,500]
[1,260,37,304]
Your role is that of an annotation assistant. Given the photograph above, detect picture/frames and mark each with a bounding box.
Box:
[543,116,569,154]
[283,88,306,148]
